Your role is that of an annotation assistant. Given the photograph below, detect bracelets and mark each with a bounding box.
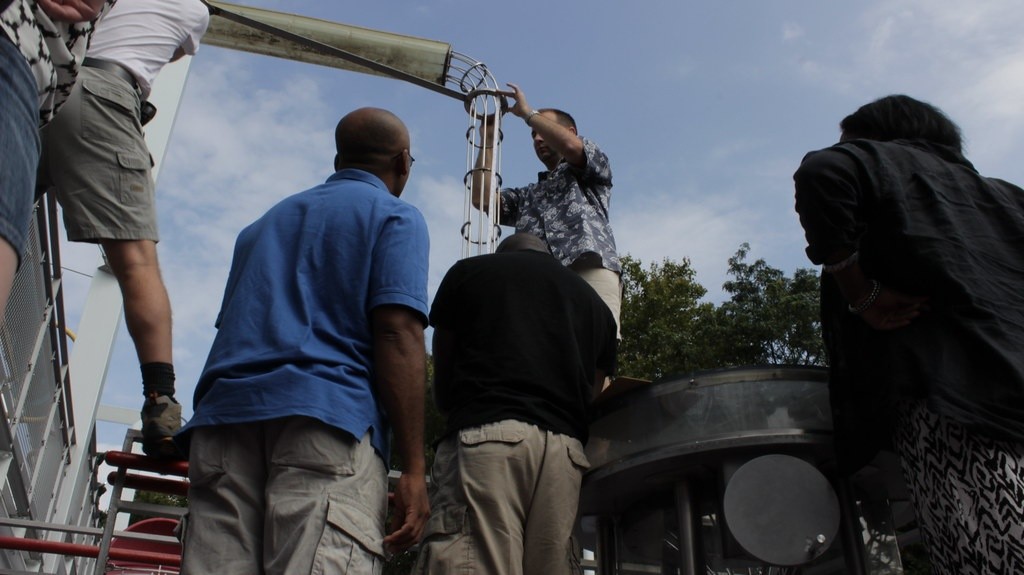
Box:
[525,111,539,124]
[848,280,880,314]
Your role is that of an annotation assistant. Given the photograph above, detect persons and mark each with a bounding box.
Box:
[176,107,430,575]
[792,95,1023,575]
[473,83,622,344]
[0,0,212,442]
[416,233,617,575]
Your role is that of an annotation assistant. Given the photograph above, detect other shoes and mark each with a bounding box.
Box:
[141,396,182,475]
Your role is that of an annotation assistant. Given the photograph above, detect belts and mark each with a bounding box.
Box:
[81,57,137,90]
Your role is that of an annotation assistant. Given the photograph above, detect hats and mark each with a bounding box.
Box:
[496,233,550,255]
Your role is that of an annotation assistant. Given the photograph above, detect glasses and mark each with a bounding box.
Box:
[391,152,415,167]
